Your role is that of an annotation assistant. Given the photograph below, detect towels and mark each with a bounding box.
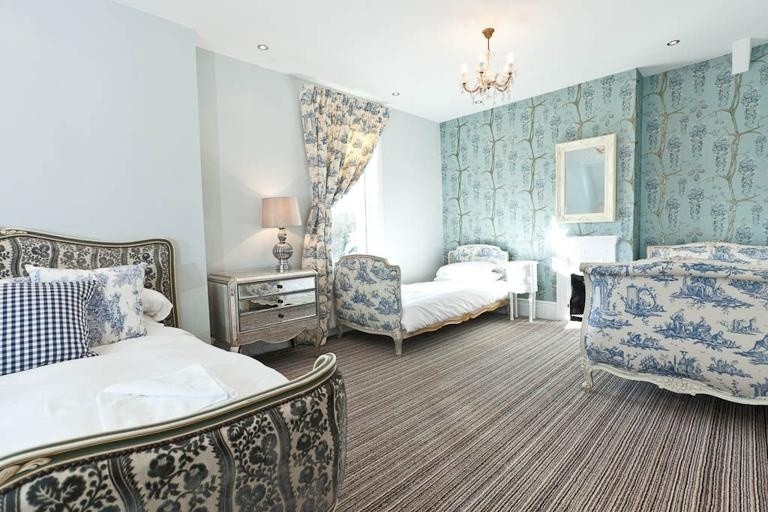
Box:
[94,362,237,435]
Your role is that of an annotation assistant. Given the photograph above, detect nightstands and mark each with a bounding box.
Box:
[506,255,540,325]
[568,272,584,323]
[205,261,325,360]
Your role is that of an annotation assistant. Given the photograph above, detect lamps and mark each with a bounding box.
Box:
[260,194,302,271]
[460,25,518,108]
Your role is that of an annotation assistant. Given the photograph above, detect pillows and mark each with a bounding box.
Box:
[19,260,152,350]
[0,279,102,374]
[433,271,503,283]
[435,258,495,278]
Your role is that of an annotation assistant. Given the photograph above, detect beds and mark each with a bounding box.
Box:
[575,240,767,410]
[0,221,351,512]
[330,241,518,356]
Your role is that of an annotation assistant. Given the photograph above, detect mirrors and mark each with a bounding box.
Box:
[553,133,619,226]
